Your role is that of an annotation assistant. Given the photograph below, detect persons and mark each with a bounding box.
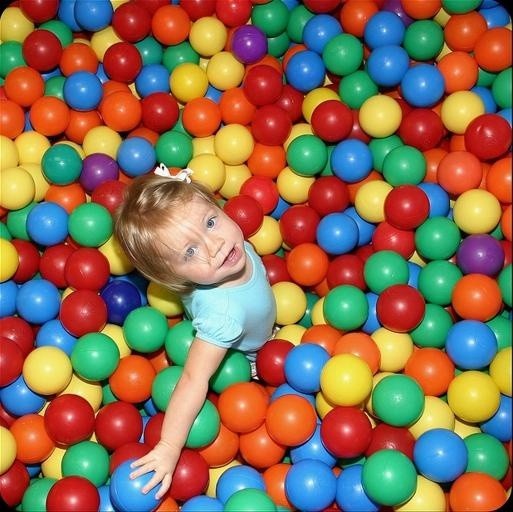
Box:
[112,165,277,500]
